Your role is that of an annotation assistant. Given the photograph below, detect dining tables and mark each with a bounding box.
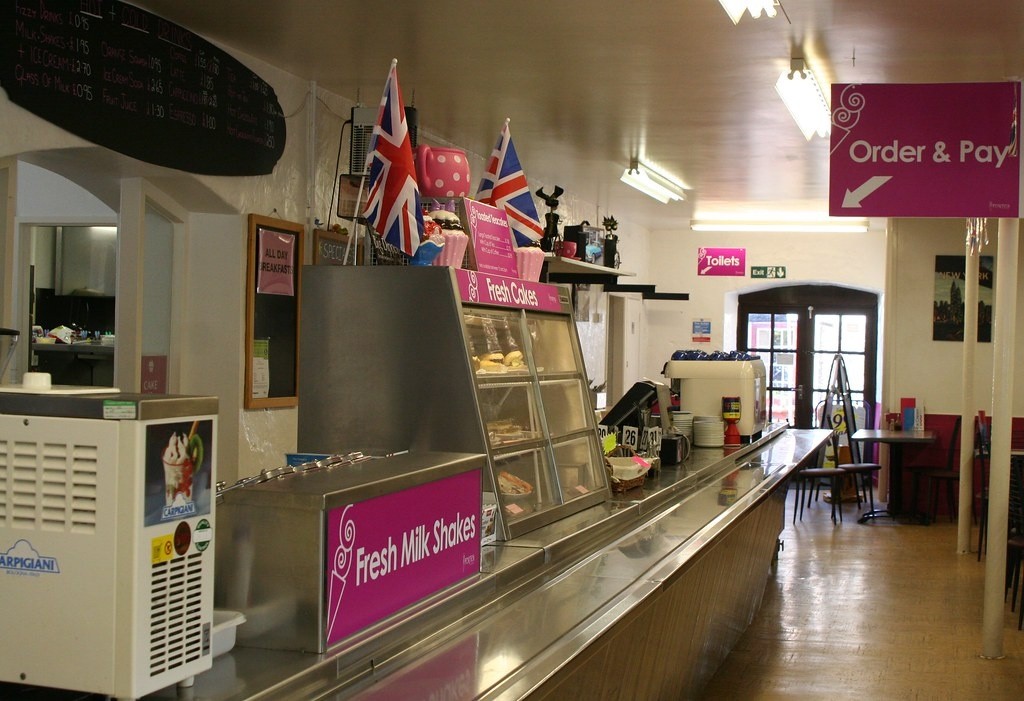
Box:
[975,448,1024,519]
[851,428,936,525]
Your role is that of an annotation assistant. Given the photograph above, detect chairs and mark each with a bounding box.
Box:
[786,416,882,525]
[904,413,1024,631]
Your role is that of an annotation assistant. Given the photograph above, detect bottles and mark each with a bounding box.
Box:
[890,419,894,431]
[895,414,902,430]
[646,441,660,474]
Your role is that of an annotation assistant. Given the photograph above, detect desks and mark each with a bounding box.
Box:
[31,339,114,386]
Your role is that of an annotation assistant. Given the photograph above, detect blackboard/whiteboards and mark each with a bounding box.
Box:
[245,211,306,411]
[312,227,366,270]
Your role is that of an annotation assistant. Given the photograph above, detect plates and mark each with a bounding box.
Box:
[509,370,529,375]
[671,416,724,447]
[486,372,508,376]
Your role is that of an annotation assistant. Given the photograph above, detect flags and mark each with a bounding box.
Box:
[472,124,544,247]
[363,67,425,255]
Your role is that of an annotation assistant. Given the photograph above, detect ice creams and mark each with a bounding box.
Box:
[161,431,193,507]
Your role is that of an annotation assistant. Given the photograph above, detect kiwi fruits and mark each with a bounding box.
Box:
[188,433,203,475]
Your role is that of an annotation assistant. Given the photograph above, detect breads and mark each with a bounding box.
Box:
[473,351,523,373]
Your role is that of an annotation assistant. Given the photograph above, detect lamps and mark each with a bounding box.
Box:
[772,57,831,142]
[717,0,781,26]
[689,201,869,234]
[619,160,687,204]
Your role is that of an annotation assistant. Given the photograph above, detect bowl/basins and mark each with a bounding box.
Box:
[501,491,530,498]
[37,337,56,344]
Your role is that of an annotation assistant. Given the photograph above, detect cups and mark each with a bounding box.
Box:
[556,242,577,258]
[412,145,471,198]
[163,460,194,507]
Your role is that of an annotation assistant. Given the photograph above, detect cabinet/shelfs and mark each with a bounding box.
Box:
[300,262,615,543]
